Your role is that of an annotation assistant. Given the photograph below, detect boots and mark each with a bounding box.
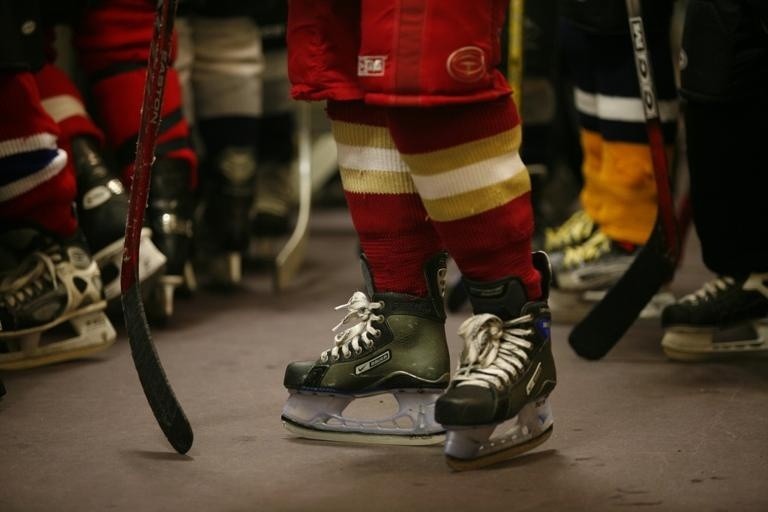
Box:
[544,231,641,295]
[531,212,599,255]
[139,136,297,287]
[432,248,558,430]
[282,248,452,393]
[57,134,133,256]
[0,197,108,340]
[658,254,768,329]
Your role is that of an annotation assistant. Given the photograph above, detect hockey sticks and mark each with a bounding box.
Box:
[120,1,193,454]
[275,99,311,294]
[568,0,682,360]
